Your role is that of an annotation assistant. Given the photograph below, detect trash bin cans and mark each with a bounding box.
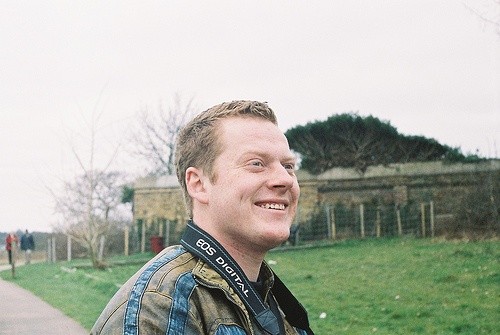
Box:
[151,237,163,254]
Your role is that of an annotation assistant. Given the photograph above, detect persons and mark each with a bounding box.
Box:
[149,237,164,254]
[5,229,35,264]
[89,100,314,335]
